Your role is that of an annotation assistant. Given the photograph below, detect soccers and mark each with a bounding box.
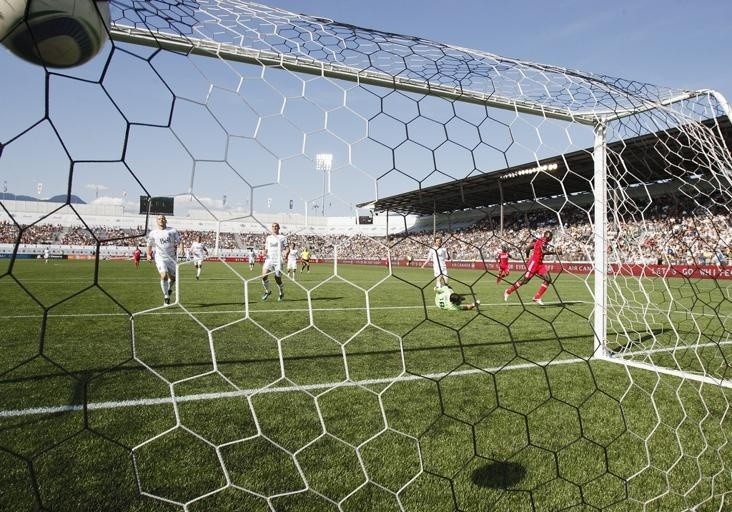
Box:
[0,0,111,68]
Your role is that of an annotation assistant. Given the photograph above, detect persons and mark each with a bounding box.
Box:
[187,235,210,280]
[299,246,311,274]
[244,249,258,272]
[286,240,300,282]
[420,237,451,287]
[145,213,183,309]
[495,245,523,284]
[433,274,482,311]
[0,190,731,263]
[259,221,291,302]
[503,229,563,307]
[132,245,143,270]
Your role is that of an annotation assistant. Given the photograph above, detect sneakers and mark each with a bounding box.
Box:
[278,293,284,302]
[164,298,170,305]
[262,291,272,300]
[168,290,172,295]
[504,289,510,302]
[196,277,200,280]
[532,297,544,305]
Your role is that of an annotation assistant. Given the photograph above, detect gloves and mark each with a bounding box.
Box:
[472,300,480,307]
